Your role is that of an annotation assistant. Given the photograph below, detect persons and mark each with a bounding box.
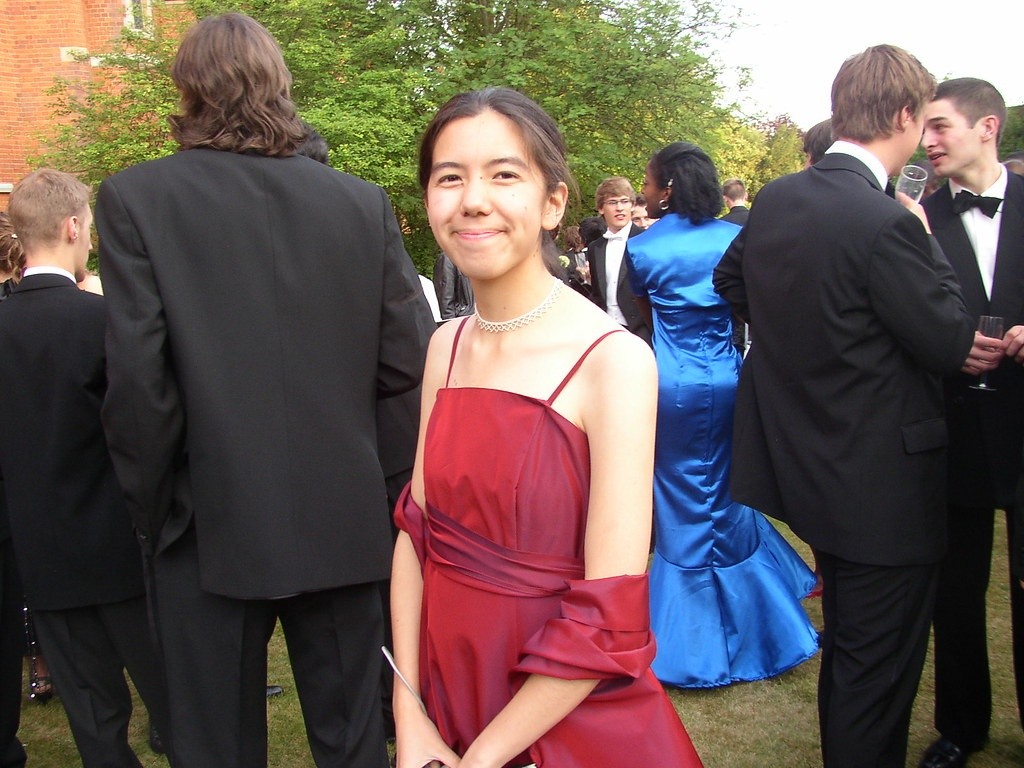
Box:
[380,87,702,768]
[95,7,437,766]
[914,76,1024,768]
[0,166,139,768]
[624,142,818,689]
[716,44,974,768]
[1,10,1024,768]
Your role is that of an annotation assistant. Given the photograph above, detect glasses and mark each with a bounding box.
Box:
[602,200,631,206]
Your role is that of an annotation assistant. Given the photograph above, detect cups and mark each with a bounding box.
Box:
[895,165,928,202]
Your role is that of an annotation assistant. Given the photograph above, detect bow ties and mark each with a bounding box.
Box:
[952,189,1004,219]
[602,234,624,242]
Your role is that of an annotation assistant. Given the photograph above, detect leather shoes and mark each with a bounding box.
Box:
[918,733,990,768]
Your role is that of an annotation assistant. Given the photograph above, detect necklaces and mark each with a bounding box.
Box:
[469,282,564,331]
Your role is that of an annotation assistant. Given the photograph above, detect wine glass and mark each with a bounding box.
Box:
[575,253,591,284]
[969,315,1004,391]
